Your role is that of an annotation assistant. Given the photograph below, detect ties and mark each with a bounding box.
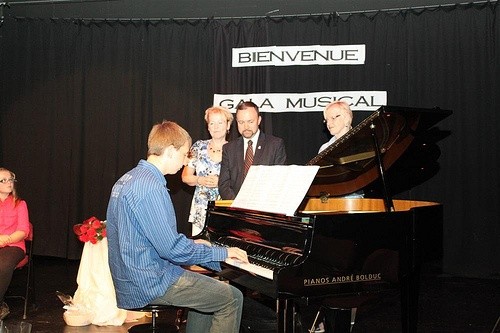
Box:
[241,140,253,183]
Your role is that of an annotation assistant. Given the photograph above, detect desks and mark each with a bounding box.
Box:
[64,236,152,327]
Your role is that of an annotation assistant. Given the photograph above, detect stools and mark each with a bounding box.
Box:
[125,304,179,333]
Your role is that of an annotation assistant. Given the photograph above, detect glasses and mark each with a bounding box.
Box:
[208,120,227,128]
[0,178,15,184]
[323,114,341,123]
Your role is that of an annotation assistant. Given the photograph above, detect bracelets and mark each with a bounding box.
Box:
[197,178,201,185]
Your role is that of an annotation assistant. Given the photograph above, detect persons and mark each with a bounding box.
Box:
[0,167,30,319]
[216,102,286,200]
[181,105,233,238]
[107,119,250,333]
[318,101,354,153]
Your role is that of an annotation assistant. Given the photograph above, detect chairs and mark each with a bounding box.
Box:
[1,223,33,319]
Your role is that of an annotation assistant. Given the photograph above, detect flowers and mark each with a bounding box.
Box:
[72,217,107,244]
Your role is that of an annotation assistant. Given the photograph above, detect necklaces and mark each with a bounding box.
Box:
[209,144,222,153]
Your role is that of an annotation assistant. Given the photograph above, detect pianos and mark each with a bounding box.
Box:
[180,105,453,333]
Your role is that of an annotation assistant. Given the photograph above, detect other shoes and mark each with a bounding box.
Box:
[308,321,326,333]
[0,302,10,320]
